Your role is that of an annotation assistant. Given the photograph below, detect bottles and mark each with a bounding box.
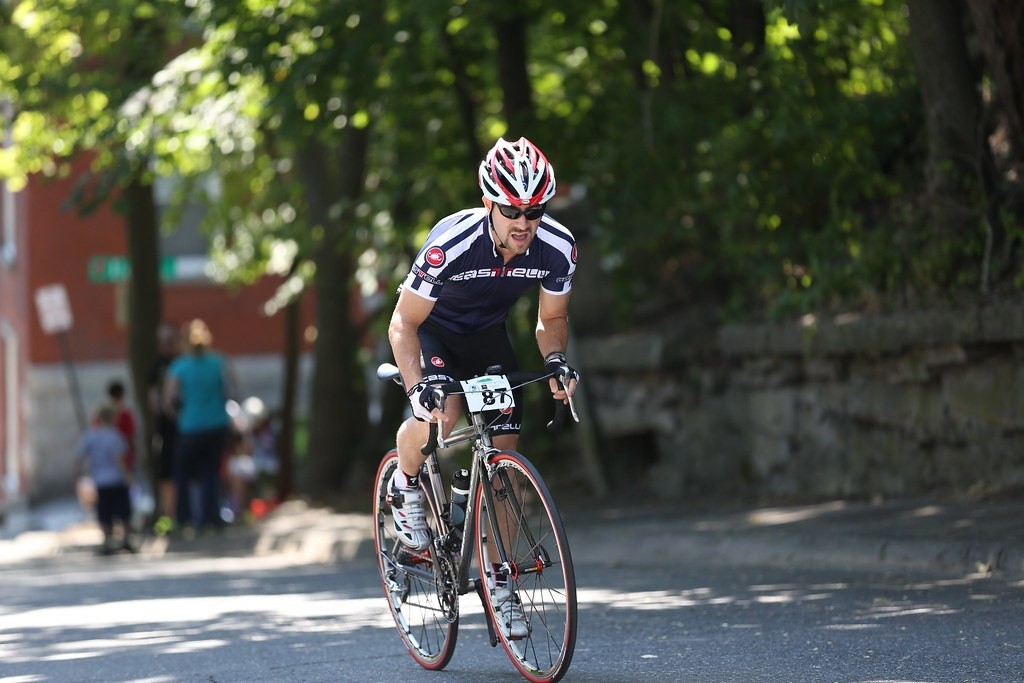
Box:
[449,468,469,533]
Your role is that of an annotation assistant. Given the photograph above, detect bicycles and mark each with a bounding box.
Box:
[371,359,579,683]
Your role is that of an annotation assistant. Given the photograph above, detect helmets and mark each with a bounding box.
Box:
[478,138,556,206]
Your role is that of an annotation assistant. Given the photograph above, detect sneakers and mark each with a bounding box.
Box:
[386,468,431,554]
[483,565,532,642]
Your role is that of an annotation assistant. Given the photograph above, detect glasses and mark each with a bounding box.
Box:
[493,202,547,220]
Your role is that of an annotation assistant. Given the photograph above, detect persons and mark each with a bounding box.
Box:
[387,134,584,642]
[67,407,140,557]
[161,318,234,533]
[92,381,141,489]
[145,324,199,542]
